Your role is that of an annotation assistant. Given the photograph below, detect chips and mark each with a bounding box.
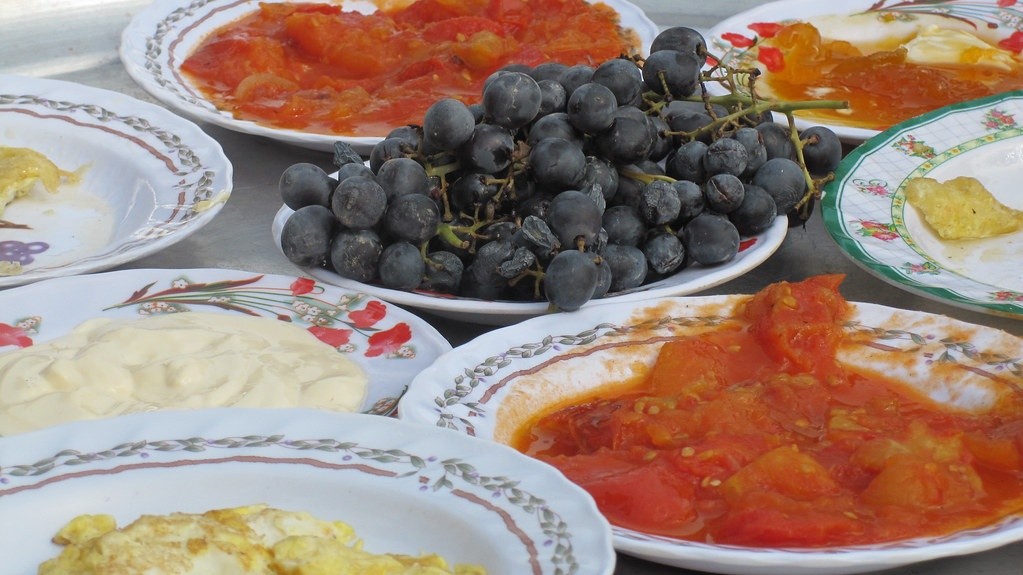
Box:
[904,176,1023,240]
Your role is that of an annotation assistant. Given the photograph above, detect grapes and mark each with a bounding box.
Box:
[279,27,844,310]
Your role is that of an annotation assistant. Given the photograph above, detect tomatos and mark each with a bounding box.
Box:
[179,0,624,133]
[545,270,1023,553]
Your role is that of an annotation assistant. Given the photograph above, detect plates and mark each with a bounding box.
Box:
[269,163,789,326]
[0,268,455,470]
[0,405,617,575]
[688,0,1022,149]
[398,294,1023,575]
[121,0,663,158]
[0,73,234,287]
[818,90,1022,323]
